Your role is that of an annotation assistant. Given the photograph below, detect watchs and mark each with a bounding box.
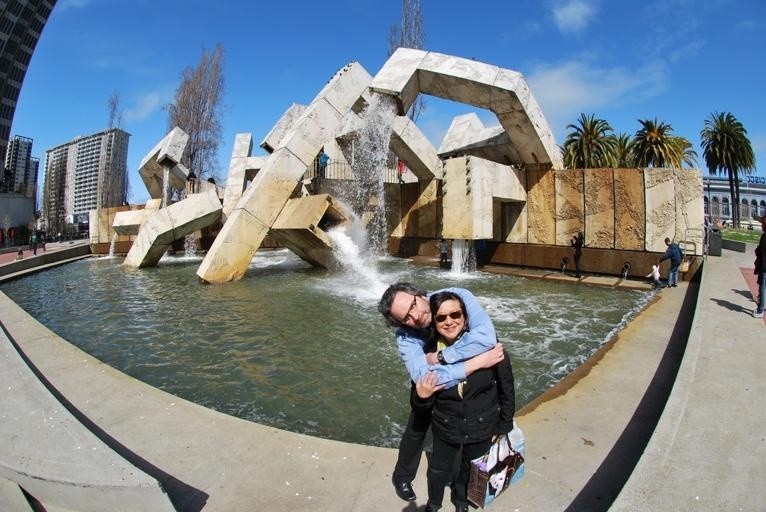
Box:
[436,350,448,367]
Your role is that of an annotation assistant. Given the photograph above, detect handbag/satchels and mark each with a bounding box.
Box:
[466,423,526,510]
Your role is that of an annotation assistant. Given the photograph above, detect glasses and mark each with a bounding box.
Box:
[401,295,418,326]
[434,311,463,323]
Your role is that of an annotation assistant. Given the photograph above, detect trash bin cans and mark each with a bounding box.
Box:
[708,228,721,256]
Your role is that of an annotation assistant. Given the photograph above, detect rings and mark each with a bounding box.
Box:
[431,383,436,388]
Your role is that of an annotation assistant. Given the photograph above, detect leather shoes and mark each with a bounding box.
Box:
[391,477,416,501]
[425,502,442,512]
[455,506,468,512]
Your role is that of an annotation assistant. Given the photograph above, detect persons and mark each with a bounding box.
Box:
[31,231,37,255]
[381,278,505,503]
[414,290,516,511]
[571,232,584,277]
[439,239,448,262]
[39,234,46,251]
[318,150,329,178]
[705,215,708,244]
[646,260,663,287]
[397,158,405,184]
[752,216,766,317]
[186,169,196,193]
[659,237,684,288]
